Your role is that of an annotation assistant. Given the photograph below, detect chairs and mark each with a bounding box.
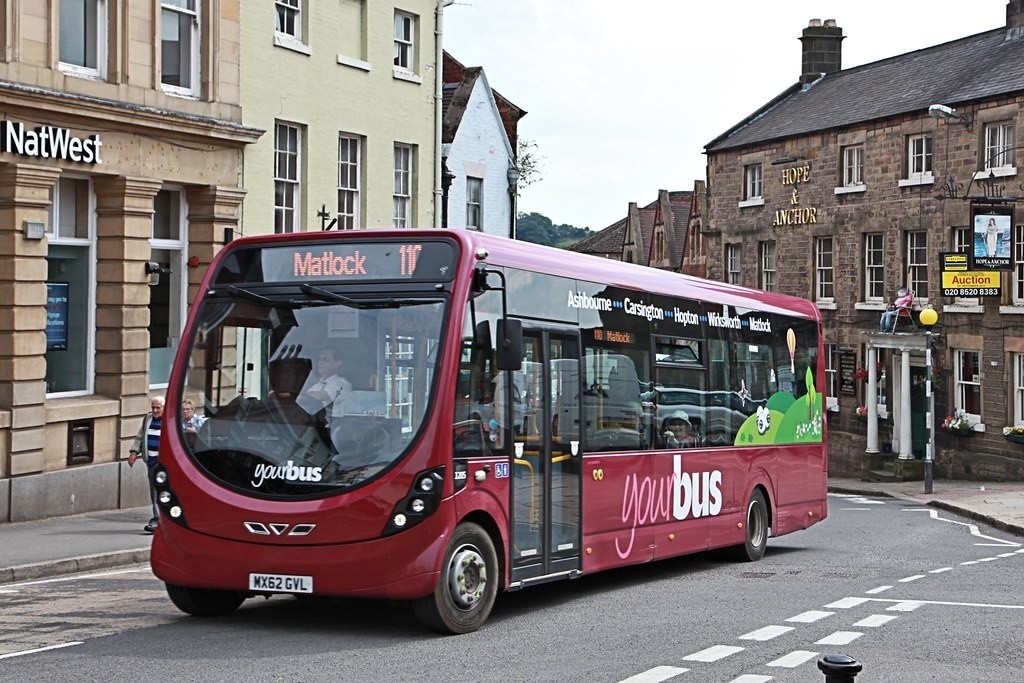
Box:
[881,290,919,334]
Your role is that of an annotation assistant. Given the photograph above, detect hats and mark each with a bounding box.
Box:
[620,402,644,417]
[666,410,692,431]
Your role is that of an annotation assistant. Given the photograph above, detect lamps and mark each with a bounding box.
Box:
[929,103,972,125]
[917,303,941,336]
[144,260,174,274]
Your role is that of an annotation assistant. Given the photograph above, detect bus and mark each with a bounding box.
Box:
[150,226,830,635]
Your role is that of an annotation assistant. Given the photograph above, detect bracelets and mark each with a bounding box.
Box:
[130,452,138,459]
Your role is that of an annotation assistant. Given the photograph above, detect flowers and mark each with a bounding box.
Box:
[853,361,886,383]
[856,406,867,416]
[941,405,972,430]
[918,367,947,386]
[1002,422,1024,436]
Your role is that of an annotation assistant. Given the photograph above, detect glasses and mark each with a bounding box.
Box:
[672,423,684,427]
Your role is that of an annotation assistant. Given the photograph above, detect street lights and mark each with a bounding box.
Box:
[919,308,939,495]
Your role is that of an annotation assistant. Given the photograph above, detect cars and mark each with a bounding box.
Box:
[650,385,768,446]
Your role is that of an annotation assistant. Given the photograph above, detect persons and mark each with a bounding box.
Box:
[619,402,697,449]
[982,219,999,258]
[268,345,353,430]
[128,396,165,531]
[879,286,912,332]
[181,398,204,434]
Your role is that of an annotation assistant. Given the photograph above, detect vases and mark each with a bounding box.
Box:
[866,376,880,385]
[858,414,867,421]
[932,383,940,391]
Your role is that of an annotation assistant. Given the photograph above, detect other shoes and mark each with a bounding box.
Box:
[144,521,158,532]
